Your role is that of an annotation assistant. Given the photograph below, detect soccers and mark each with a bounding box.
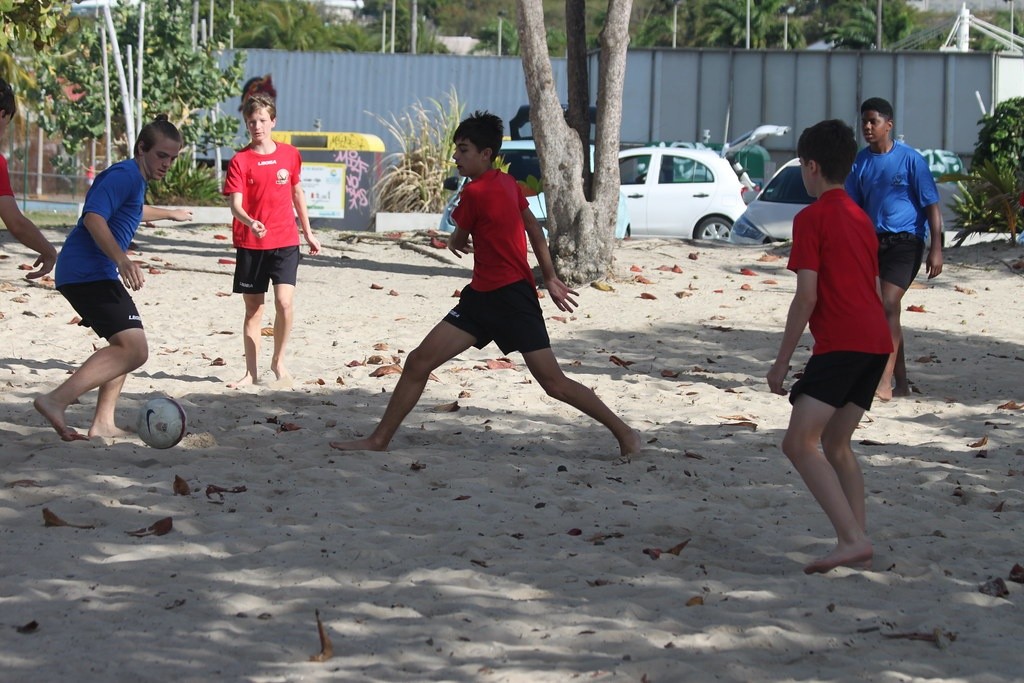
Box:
[135,398,188,449]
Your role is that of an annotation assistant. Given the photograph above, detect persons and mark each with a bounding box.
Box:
[767,119,895,573]
[0,77,55,282]
[327,111,646,468]
[848,95,946,401]
[224,93,322,387]
[26,112,197,441]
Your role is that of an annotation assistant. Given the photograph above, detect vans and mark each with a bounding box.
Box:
[730,146,971,258]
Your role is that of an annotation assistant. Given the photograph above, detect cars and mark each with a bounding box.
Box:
[437,138,631,242]
[580,116,793,244]
